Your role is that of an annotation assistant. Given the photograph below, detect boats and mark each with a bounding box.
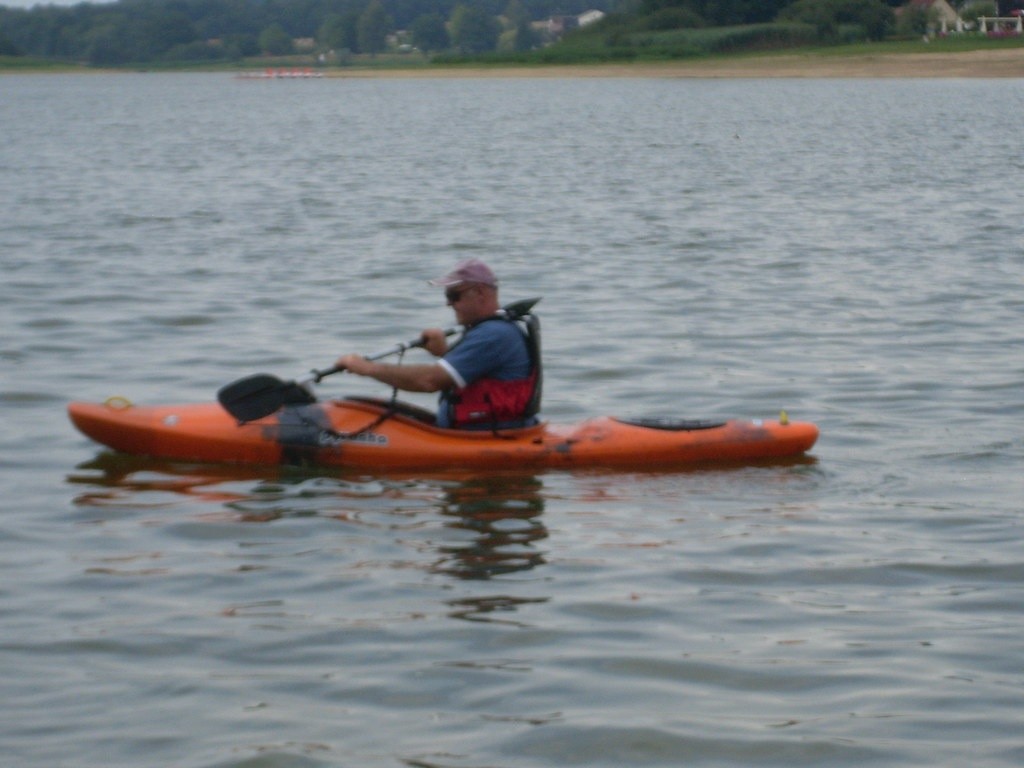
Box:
[68,394,821,473]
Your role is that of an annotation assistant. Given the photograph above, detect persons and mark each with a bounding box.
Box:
[339,258,544,431]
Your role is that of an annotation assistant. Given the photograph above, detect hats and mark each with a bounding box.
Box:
[428,259,500,288]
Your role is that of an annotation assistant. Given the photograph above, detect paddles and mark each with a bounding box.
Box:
[217,294,546,426]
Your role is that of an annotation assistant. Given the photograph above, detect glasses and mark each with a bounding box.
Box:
[446,284,474,299]
